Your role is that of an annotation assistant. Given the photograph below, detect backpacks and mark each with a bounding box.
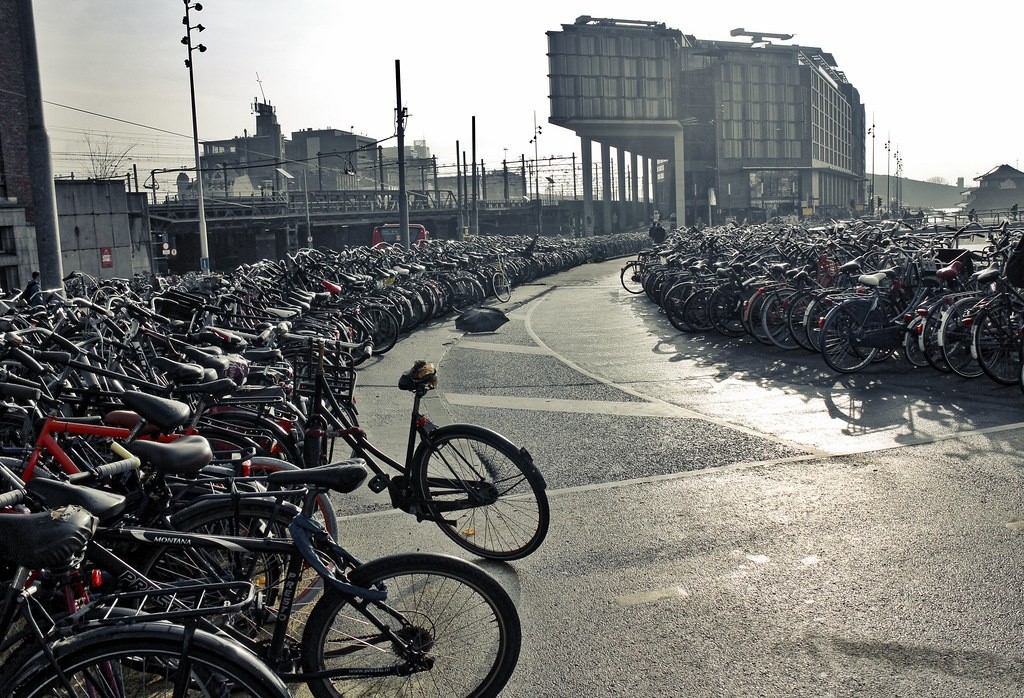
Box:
[19,282,39,305]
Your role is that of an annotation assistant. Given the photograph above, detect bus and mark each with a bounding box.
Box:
[373,224,429,249]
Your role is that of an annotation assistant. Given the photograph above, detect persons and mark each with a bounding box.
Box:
[968,208,975,222]
[652,222,665,244]
[1010,203,1019,221]
[903,207,924,227]
[649,222,658,246]
[26,270,43,307]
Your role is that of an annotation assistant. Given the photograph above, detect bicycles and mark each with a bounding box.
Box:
[0,233,657,698]
[621,219,1024,391]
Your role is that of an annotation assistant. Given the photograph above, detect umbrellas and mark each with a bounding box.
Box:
[455,306,510,333]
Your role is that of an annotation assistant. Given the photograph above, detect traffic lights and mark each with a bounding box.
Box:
[879,198,882,206]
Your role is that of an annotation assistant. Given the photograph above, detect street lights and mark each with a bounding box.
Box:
[894,146,903,211]
[884,129,890,213]
[529,109,543,235]
[181,0,211,275]
[868,112,876,215]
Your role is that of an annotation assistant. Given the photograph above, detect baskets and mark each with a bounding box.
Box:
[923,248,973,283]
[158,289,206,325]
